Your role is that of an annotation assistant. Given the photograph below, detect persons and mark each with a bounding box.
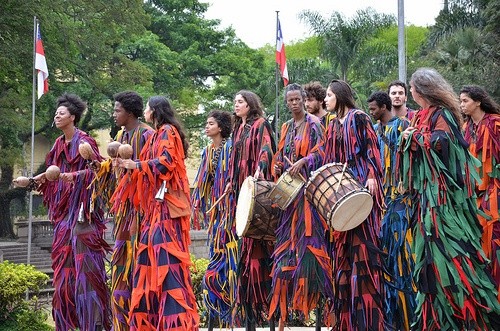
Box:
[12,67,500,331]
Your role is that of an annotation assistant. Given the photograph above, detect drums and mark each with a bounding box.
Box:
[235,176,282,242]
[305,163,373,232]
[267,171,304,210]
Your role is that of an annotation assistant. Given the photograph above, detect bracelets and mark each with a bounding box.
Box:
[135,162,138,169]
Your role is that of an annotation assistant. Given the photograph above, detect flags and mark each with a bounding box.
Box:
[275,18,289,86]
[35,19,50,100]
